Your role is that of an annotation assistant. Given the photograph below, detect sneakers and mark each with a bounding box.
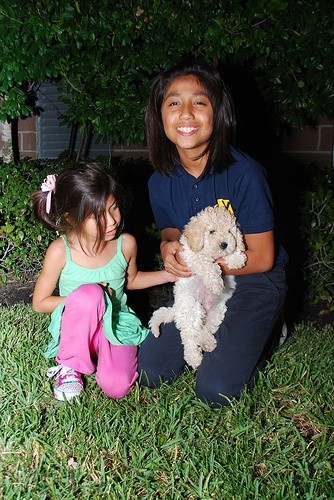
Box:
[47,364,84,401]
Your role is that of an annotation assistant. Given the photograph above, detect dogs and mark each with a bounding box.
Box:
[148,203,247,371]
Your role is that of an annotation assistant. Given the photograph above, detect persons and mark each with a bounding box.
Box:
[139,63,290,406]
[30,162,178,400]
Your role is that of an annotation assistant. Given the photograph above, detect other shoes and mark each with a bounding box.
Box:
[279,314,287,346]
